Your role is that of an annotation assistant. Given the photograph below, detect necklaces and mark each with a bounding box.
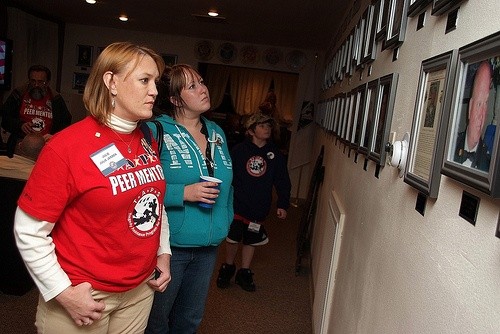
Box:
[112,128,136,153]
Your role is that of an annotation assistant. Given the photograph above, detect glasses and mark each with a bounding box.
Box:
[31,80,48,85]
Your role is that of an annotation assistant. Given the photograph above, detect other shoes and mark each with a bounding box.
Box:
[235,266,257,292]
[217,263,237,289]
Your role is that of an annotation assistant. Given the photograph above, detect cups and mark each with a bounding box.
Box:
[197,175,222,209]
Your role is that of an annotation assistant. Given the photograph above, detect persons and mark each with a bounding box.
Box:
[14,42,172,334]
[424,82,437,127]
[454,60,492,172]
[215,113,292,292]
[145,63,234,334]
[0,65,72,141]
[257,91,280,139]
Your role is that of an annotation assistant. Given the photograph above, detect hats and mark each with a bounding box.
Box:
[243,113,275,130]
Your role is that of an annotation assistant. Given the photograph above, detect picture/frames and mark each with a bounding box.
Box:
[316,0,500,199]
[72,44,177,91]
